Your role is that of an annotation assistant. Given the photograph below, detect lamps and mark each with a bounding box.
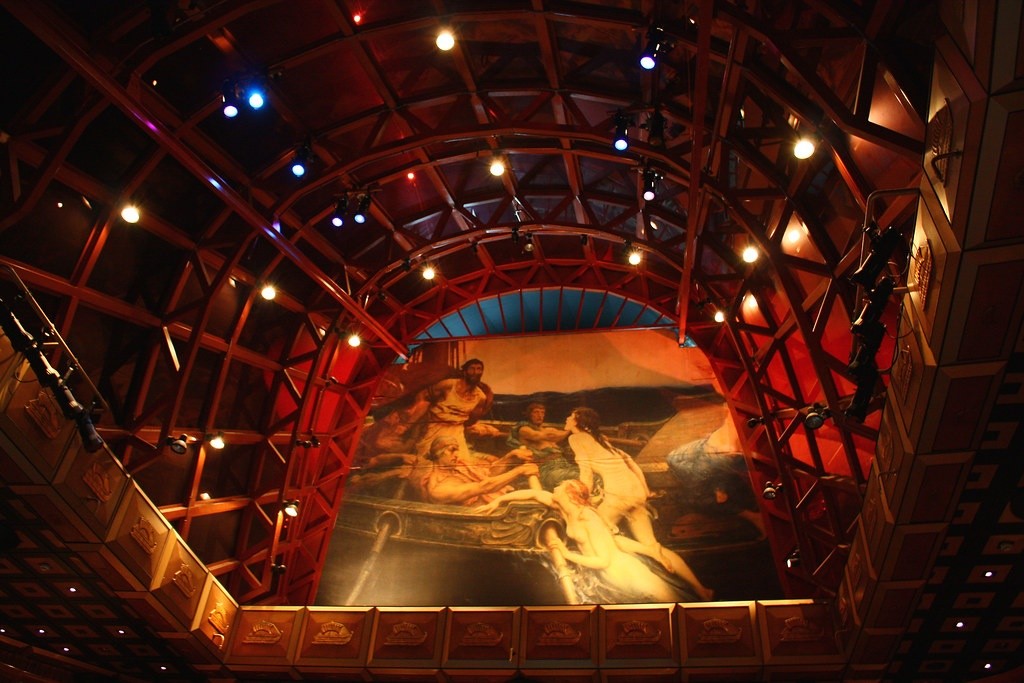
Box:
[437,19,454,50]
[851,276,897,335]
[84,195,372,518]
[612,112,632,150]
[646,115,667,147]
[748,386,873,571]
[511,229,518,243]
[524,233,534,250]
[248,62,290,112]
[404,258,410,271]
[218,74,244,117]
[641,169,663,201]
[290,143,309,177]
[623,244,638,262]
[793,131,822,160]
[638,31,674,69]
[851,225,902,286]
[849,321,887,378]
[582,234,589,247]
